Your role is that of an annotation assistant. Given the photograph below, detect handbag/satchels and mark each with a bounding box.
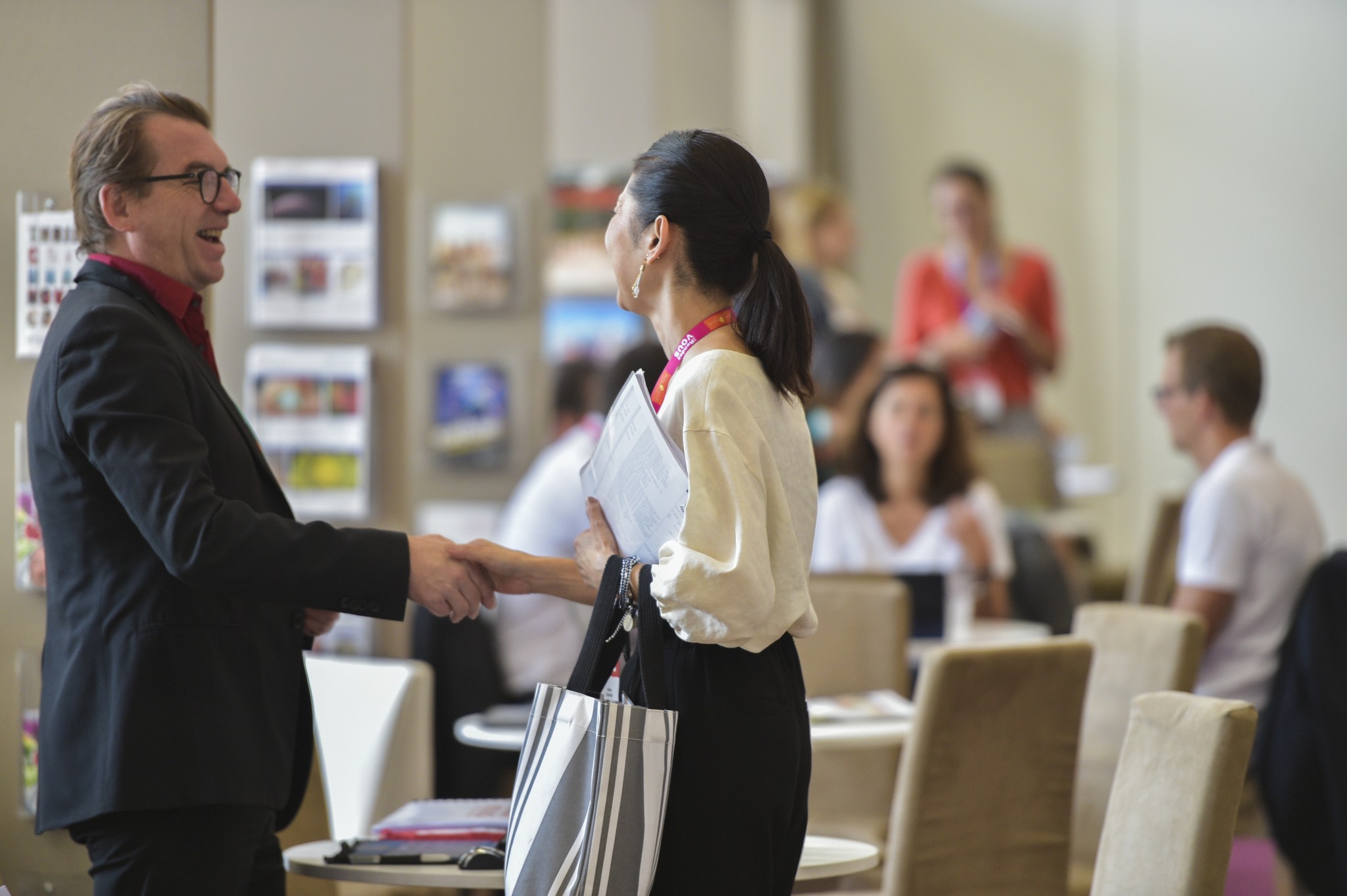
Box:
[504,554,680,895]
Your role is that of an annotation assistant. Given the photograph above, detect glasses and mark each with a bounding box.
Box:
[113,169,241,205]
[1156,380,1194,412]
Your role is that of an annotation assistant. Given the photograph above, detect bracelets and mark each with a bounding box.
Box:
[965,566,996,580]
[604,553,638,644]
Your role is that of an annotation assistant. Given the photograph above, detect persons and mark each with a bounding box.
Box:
[555,357,614,436]
[32,80,494,896]
[1155,323,1332,777]
[808,364,1017,625]
[766,182,883,486]
[486,340,677,703]
[1251,545,1347,894]
[454,128,818,896]
[887,161,1070,564]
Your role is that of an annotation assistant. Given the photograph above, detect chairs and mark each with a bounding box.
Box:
[1087,689,1259,895]
[789,570,910,704]
[872,635,1097,896]
[1062,600,1211,896]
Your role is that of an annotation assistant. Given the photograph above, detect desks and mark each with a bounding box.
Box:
[449,698,912,829]
[906,618,1046,676]
[282,826,883,896]
